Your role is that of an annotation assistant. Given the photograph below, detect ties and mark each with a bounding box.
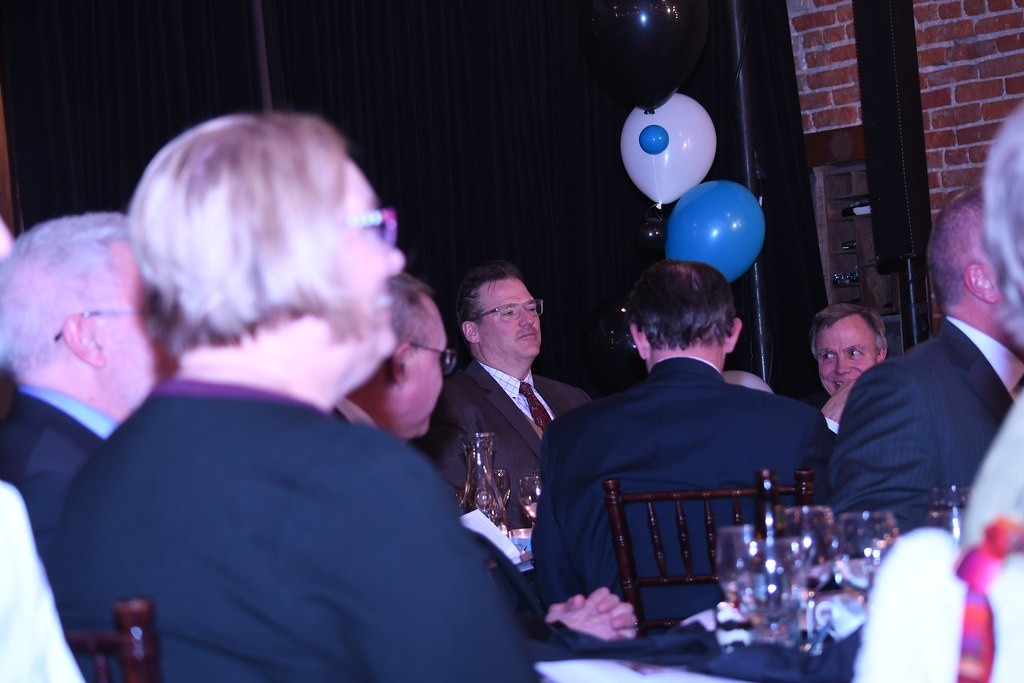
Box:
[519,382,551,432]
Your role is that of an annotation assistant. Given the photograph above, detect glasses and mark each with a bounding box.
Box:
[53,293,160,343]
[341,204,398,247]
[408,341,456,375]
[469,298,543,321]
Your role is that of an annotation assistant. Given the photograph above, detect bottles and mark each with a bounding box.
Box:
[458,430,509,537]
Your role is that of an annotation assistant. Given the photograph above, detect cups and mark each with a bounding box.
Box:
[493,468,511,505]
[518,476,541,524]
[714,483,971,650]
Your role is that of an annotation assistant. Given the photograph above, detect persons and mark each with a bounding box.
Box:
[527,260,832,634]
[811,99,1024,683]
[0,113,640,683]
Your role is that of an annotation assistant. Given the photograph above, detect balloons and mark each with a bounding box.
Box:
[576,0,710,113]
[722,371,774,395]
[620,93,716,209]
[664,180,766,284]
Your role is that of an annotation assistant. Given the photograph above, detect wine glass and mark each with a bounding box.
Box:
[777,505,835,646]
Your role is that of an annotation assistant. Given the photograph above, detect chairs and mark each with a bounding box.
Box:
[602,469,814,636]
